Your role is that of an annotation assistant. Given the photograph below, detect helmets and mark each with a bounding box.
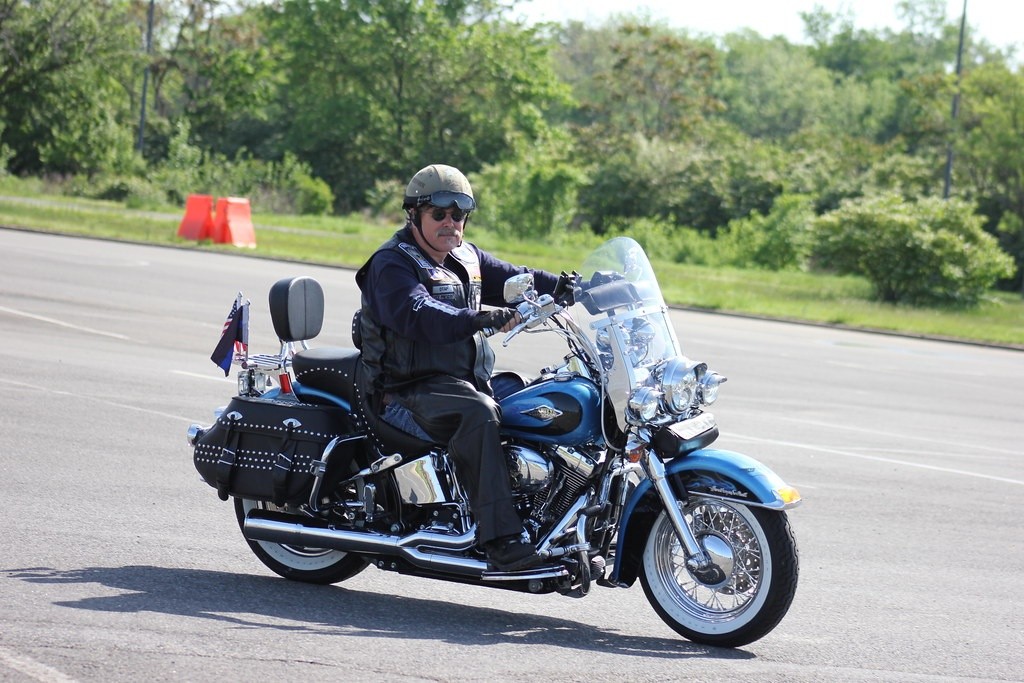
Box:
[404,164,477,212]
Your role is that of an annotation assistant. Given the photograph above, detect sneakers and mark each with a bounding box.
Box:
[488,538,541,572]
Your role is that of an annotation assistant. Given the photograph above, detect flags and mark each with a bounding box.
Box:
[210,302,250,378]
[221,291,248,354]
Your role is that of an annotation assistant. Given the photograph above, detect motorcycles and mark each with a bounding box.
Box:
[187,235,800,647]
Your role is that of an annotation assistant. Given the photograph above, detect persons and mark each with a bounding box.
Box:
[356,163,623,579]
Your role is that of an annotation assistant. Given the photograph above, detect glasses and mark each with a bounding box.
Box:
[421,209,465,223]
[418,190,476,211]
